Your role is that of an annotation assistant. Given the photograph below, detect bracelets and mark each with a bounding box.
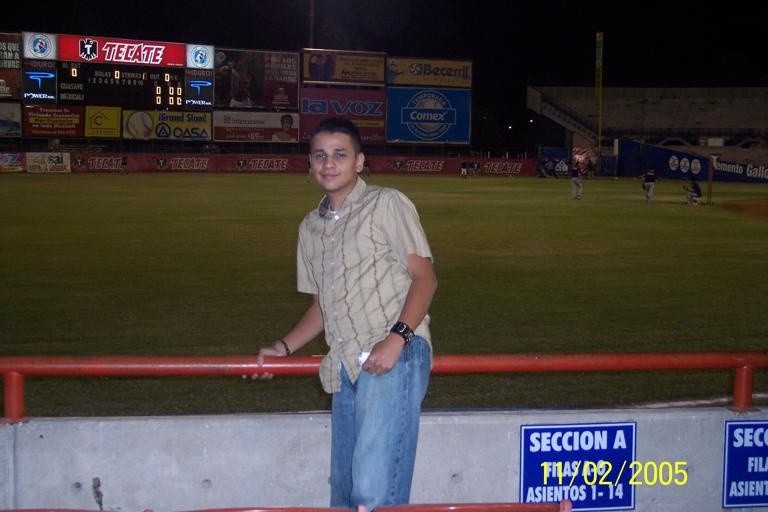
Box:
[278,338,291,357]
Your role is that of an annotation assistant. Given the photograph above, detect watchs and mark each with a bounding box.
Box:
[389,319,416,344]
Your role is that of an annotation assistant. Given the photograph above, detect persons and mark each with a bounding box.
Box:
[641,166,658,203]
[472,160,479,176]
[461,159,468,176]
[240,114,439,510]
[361,159,371,177]
[383,61,405,85]
[269,114,298,142]
[304,161,313,179]
[569,160,583,200]
[219,59,255,110]
[543,160,558,178]
[586,159,595,178]
[119,155,129,176]
[685,177,703,206]
